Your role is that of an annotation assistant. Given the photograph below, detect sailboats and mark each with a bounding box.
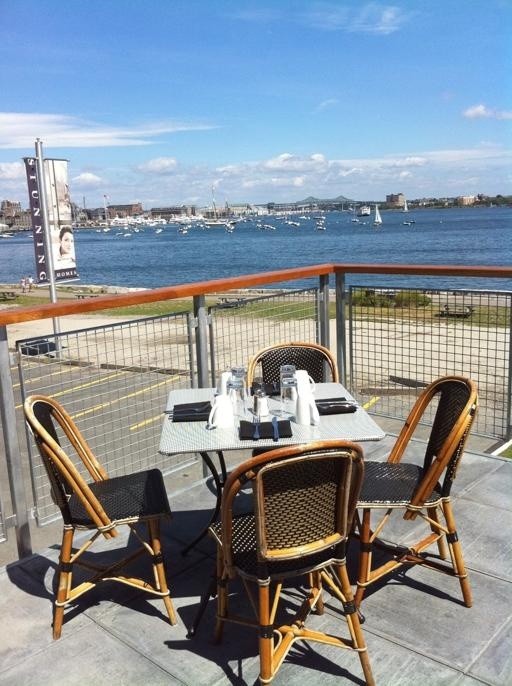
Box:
[373,204,383,226]
[351,207,359,223]
[169,196,327,234]
[401,199,411,213]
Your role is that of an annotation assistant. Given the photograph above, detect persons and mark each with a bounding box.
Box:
[58,226,76,261]
[21,276,28,293]
[26,275,35,292]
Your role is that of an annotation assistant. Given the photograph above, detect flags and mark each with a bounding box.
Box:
[43,157,81,283]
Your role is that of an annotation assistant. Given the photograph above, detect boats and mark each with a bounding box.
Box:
[410,220,415,223]
[403,221,411,225]
[1,190,168,240]
[357,205,370,216]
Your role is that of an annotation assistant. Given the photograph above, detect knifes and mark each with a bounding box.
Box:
[169,413,210,418]
[315,401,354,404]
[272,416,279,440]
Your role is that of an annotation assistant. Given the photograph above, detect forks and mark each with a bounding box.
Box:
[163,403,210,414]
[252,413,261,439]
[318,404,354,409]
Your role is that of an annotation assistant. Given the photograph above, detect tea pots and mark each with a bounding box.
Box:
[208,392,236,431]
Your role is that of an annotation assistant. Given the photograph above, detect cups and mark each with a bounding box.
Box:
[280,364,298,418]
[225,367,246,420]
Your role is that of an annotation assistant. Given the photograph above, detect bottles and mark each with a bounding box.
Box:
[253,388,270,417]
